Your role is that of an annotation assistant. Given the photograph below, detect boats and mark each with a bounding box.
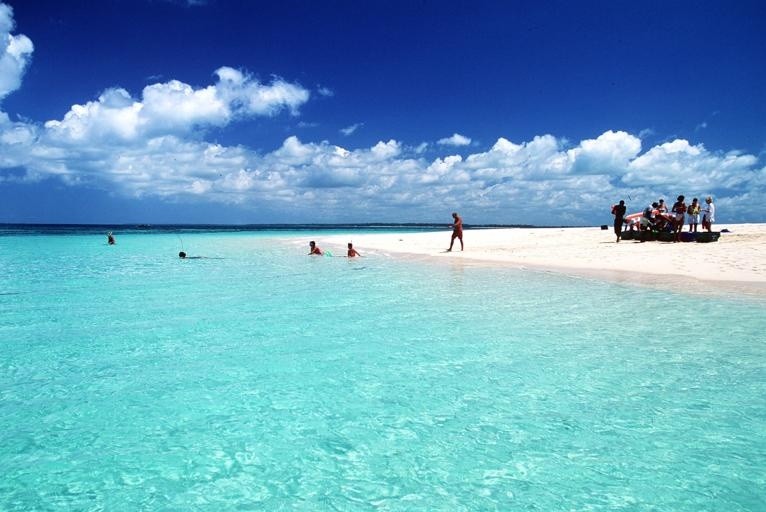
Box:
[620,230,720,243]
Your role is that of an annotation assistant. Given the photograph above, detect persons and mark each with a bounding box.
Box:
[638,195,715,243]
[107,232,116,243]
[179,251,186,258]
[308,241,321,255]
[446,212,464,251]
[348,243,360,257]
[612,200,628,243]
